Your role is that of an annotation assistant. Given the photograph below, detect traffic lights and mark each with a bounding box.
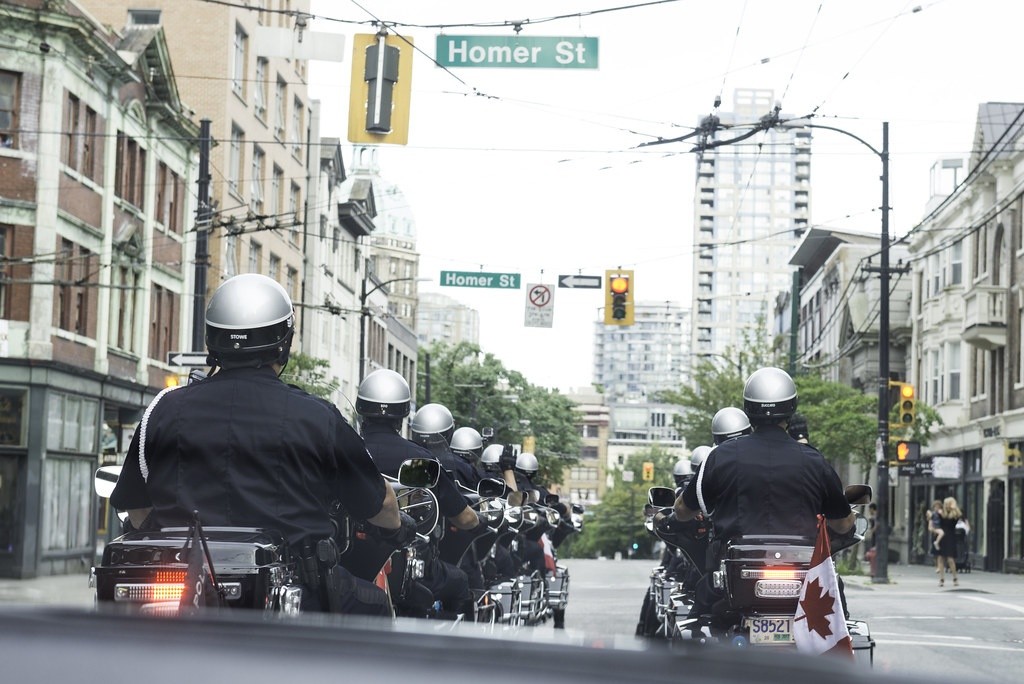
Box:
[895,441,920,461]
[605,270,634,325]
[643,462,654,481]
[900,384,915,424]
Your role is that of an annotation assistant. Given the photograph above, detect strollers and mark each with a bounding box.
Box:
[945,529,970,573]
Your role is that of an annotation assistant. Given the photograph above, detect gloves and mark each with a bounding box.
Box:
[468,512,489,535]
[668,512,701,532]
[498,442,517,473]
[363,508,417,548]
[788,412,809,440]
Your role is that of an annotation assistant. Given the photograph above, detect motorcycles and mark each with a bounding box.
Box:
[642,484,876,666]
[88,432,585,628]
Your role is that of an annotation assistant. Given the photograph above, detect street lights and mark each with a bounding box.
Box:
[696,353,742,380]
[775,123,890,584]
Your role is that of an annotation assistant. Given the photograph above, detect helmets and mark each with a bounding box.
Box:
[481,443,505,463]
[711,406,751,444]
[450,426,484,459]
[691,445,716,471]
[673,459,696,486]
[411,402,456,447]
[515,452,539,471]
[743,366,798,426]
[204,273,296,377]
[356,368,411,418]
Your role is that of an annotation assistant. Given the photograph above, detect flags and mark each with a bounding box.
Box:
[792,518,855,666]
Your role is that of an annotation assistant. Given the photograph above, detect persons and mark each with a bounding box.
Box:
[633,367,856,641]
[107,272,573,619]
[926,496,971,585]
[866,502,880,546]
